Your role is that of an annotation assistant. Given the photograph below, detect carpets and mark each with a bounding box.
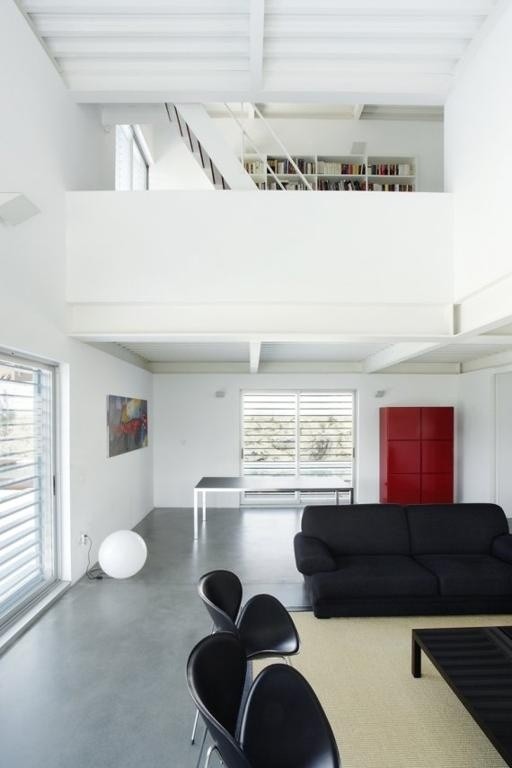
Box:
[251,610,512,768]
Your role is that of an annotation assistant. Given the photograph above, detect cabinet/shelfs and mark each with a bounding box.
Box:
[380,407,454,506]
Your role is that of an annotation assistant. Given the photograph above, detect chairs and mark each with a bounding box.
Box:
[186,635,340,768]
[190,570,301,745]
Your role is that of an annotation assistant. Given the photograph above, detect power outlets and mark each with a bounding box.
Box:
[82,534,88,545]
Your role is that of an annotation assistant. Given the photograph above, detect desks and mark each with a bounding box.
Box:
[193,476,353,539]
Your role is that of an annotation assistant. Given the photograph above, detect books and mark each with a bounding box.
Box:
[244,159,415,192]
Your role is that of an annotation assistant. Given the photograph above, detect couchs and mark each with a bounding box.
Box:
[294,503,512,619]
